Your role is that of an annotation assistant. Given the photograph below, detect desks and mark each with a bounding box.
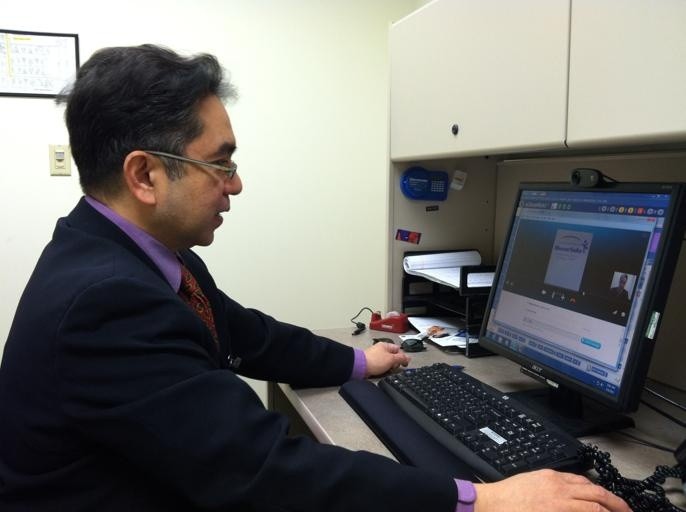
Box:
[266,321,684,510]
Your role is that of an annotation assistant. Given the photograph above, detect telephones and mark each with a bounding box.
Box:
[674,438,686,466]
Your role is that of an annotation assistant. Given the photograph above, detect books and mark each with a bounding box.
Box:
[402,250,498,290]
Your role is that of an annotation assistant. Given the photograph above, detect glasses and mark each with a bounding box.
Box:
[145,150,237,180]
[372,336,428,352]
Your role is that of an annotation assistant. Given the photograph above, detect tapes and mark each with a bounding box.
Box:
[386,311,399,318]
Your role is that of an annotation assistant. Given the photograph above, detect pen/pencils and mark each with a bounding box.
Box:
[405,365,465,372]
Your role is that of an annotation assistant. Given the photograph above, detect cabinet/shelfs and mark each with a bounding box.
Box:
[384,0,685,164]
[399,247,498,359]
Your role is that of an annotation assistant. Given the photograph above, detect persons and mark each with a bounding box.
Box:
[1,43,633,512]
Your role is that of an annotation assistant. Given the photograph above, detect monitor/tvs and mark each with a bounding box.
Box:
[478,182,686,437]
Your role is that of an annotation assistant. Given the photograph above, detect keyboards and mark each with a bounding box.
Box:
[378,363,594,483]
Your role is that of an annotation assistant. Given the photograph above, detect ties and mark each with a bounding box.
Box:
[179,263,217,346]
